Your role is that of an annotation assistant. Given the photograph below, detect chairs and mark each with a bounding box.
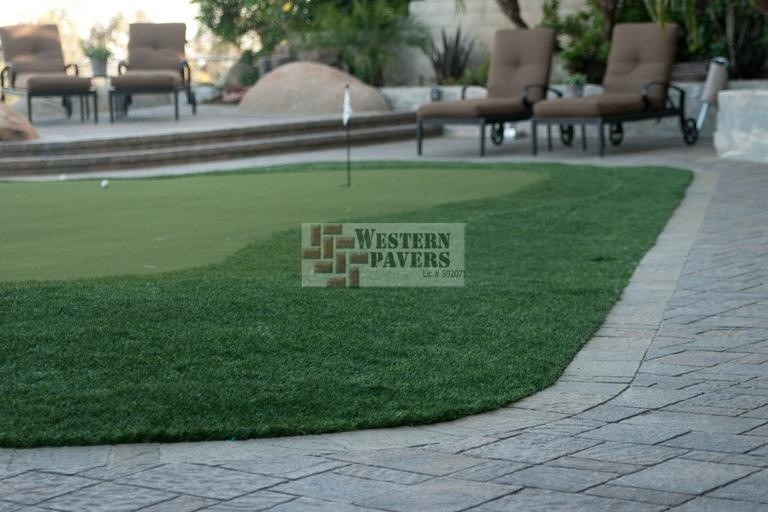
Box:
[0,22,197,122]
[416,21,698,157]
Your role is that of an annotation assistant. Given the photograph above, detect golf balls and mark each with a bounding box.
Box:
[101,180,108,187]
[59,175,65,181]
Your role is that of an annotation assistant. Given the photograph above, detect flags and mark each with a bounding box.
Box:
[343,86,352,128]
